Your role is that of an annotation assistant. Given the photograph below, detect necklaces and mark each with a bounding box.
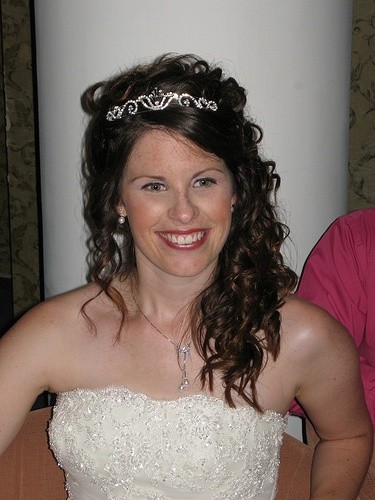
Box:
[125,269,201,391]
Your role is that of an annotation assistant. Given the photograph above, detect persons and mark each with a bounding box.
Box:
[1,47,374,500]
[287,206,373,500]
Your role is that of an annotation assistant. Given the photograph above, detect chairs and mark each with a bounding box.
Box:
[0,406,312,500]
